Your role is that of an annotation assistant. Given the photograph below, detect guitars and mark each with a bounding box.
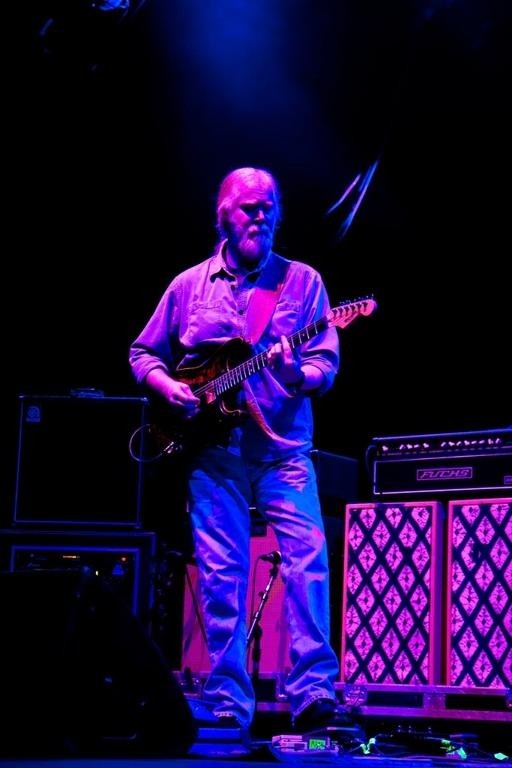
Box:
[148,295,379,454]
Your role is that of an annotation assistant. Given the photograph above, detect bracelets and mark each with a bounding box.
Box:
[285,369,305,391]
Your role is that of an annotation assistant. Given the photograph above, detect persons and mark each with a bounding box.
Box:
[128,167,360,736]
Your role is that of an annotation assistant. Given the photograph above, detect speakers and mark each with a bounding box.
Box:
[181,527,343,713]
[12,395,151,530]
[443,497,512,688]
[338,499,447,684]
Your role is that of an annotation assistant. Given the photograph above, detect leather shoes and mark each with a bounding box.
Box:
[212,711,240,727]
[295,698,354,733]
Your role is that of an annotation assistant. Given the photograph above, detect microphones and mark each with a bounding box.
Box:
[257,548,285,568]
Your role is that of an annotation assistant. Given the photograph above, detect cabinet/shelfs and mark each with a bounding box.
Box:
[332,498,512,722]
